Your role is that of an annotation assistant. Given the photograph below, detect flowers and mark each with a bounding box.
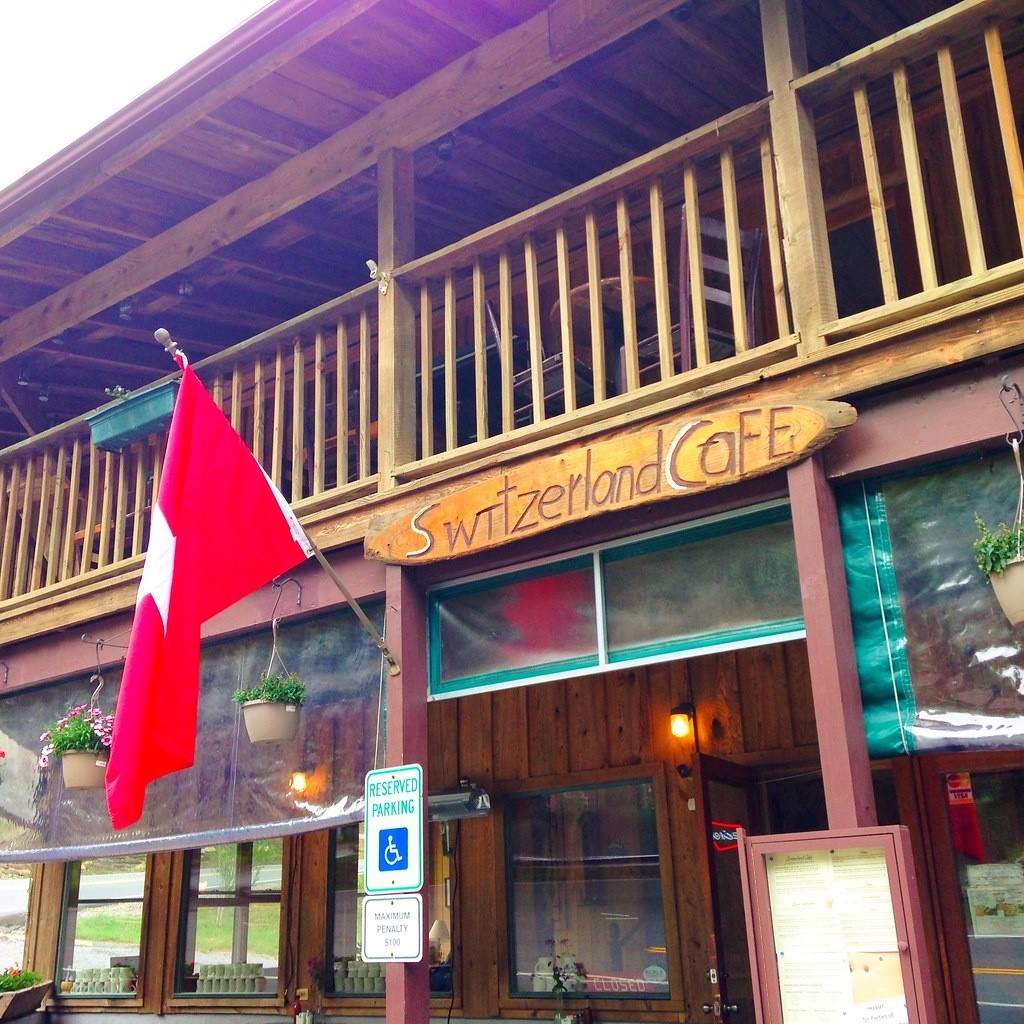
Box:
[38,701,115,767]
[544,938,569,1018]
[0,961,47,993]
[571,961,590,1008]
[104,384,133,402]
[306,955,325,1014]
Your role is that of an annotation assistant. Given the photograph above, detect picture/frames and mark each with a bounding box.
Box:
[442,821,449,856]
[445,877,450,907]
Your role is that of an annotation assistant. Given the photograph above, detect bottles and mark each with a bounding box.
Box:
[554,988,593,1024]
[292,994,301,1023]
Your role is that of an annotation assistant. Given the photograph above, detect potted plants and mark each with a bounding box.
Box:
[971,511,1024,631]
[232,668,306,747]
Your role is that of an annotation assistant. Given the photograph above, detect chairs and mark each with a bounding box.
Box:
[485,299,616,428]
[303,389,361,496]
[113,475,153,558]
[620,203,765,394]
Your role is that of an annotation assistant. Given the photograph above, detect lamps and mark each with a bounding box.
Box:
[38,384,50,401]
[671,701,694,738]
[52,336,65,345]
[289,761,316,793]
[17,372,29,386]
[119,310,132,320]
[428,919,449,961]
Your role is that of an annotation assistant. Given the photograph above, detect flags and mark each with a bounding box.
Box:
[104,351,315,834]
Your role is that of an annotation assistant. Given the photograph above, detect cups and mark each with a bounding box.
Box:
[295,1009,314,1024]
[196,963,265,993]
[60,965,139,995]
[530,956,587,991]
[334,960,386,993]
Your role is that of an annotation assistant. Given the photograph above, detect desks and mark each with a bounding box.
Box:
[75,520,115,570]
[548,276,673,396]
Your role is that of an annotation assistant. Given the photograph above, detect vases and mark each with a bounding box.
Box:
[584,1007,592,1024]
[61,749,110,791]
[313,1013,328,1024]
[0,979,53,1021]
[84,378,181,452]
[554,1015,574,1024]
